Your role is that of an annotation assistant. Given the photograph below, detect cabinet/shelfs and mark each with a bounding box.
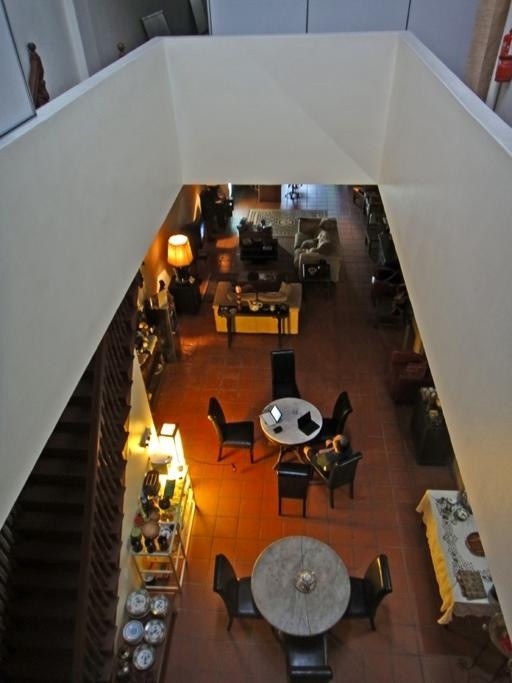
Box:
[408,378,454,469]
[199,185,235,237]
[257,184,284,204]
[167,273,201,316]
[374,238,398,268]
[146,289,183,365]
[127,462,202,601]
[137,326,170,417]
[197,235,214,267]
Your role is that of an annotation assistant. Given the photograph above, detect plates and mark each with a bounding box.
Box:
[122,591,169,671]
[455,506,469,521]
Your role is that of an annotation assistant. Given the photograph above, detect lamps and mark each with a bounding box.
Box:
[157,419,187,474]
[166,231,194,285]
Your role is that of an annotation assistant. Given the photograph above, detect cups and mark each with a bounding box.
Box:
[157,535,168,551]
[425,399,434,412]
[140,496,152,512]
[129,537,142,553]
[143,537,156,553]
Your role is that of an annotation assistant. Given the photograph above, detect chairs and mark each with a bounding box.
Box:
[317,388,353,439]
[341,553,393,633]
[370,264,409,331]
[360,188,384,219]
[274,454,315,517]
[303,441,363,509]
[212,551,264,632]
[274,625,338,681]
[386,346,430,403]
[270,347,302,400]
[352,184,367,203]
[206,395,257,465]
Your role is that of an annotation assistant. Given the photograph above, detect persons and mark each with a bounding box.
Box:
[240,271,277,290]
[303,433,352,477]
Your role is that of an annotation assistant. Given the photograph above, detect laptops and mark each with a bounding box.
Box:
[257,404,282,428]
[298,411,320,436]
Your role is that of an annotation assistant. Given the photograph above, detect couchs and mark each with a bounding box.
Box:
[210,278,303,335]
[293,213,344,285]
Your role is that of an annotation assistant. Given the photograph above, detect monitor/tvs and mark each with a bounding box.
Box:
[184,219,206,249]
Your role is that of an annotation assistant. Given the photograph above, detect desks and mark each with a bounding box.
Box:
[111,586,179,682]
[259,394,324,470]
[215,303,292,349]
[240,237,281,264]
[238,280,282,293]
[247,534,354,640]
[362,222,391,254]
[300,263,332,302]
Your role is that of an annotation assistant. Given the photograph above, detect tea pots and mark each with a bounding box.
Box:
[247,299,263,312]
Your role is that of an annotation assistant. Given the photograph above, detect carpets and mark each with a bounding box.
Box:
[201,234,297,304]
[244,207,330,239]
